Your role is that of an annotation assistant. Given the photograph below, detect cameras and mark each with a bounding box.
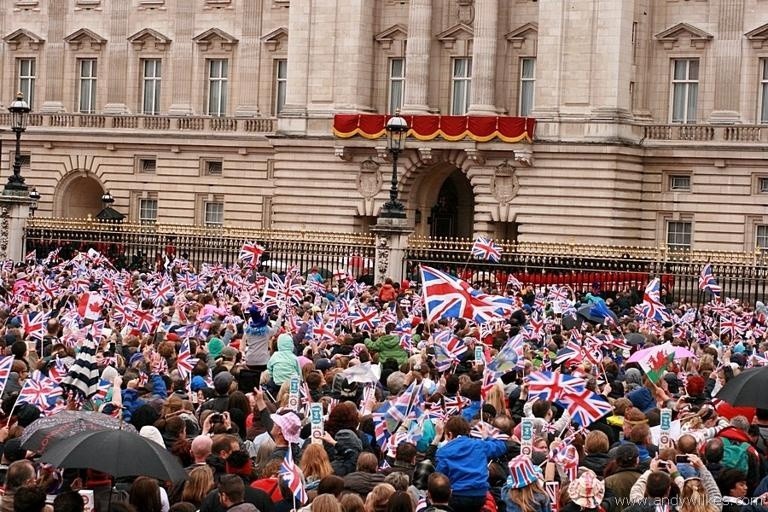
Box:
[657,461,669,469]
[685,397,696,403]
[211,412,223,423]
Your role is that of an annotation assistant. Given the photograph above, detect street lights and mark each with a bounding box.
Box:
[378,111,409,217]
[2,93,33,197]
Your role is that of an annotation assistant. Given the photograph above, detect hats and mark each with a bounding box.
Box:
[129,328,436,444]
[506,304,706,509]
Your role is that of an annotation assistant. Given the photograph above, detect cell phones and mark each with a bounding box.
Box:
[676,456,690,463]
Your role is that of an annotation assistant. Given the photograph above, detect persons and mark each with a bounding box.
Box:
[0,235,767,512]
